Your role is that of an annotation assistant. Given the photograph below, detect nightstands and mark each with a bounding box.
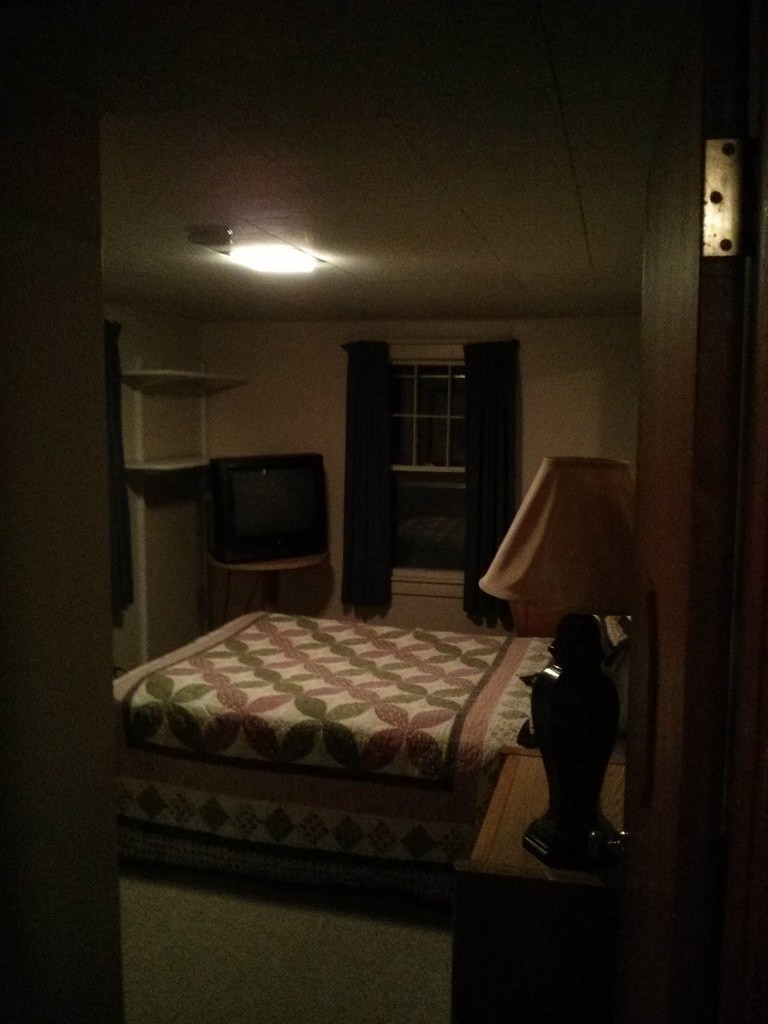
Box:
[450,746,628,1024]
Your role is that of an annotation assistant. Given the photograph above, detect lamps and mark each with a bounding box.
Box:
[476,453,644,872]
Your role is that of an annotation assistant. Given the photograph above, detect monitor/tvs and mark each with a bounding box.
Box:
[211,453,329,565]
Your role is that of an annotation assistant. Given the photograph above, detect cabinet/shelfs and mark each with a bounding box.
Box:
[123,367,329,572]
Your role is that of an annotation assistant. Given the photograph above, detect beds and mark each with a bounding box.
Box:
[112,609,630,898]
